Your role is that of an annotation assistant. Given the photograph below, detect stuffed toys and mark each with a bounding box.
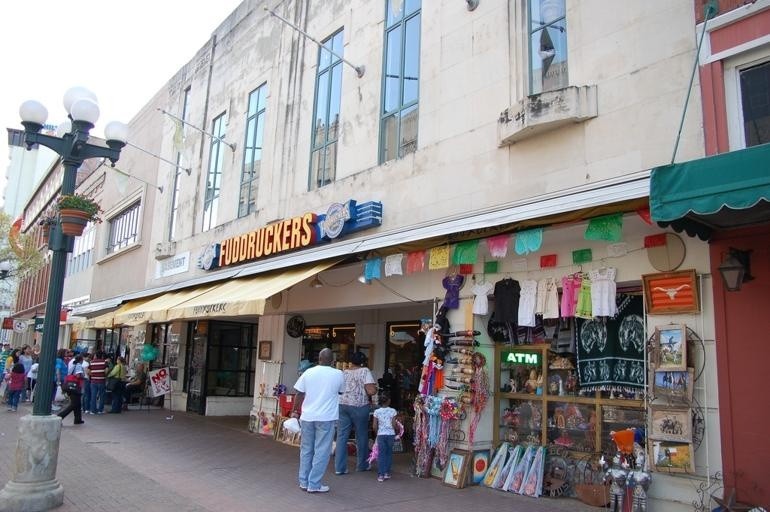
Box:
[508,377,518,394]
[536,373,543,395]
[525,370,538,395]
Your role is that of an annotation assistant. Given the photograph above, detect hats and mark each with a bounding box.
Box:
[283,417,300,433]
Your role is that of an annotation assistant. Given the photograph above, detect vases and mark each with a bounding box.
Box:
[43,227,50,243]
[61,209,90,236]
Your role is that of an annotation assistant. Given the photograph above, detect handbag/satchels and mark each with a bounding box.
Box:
[62,375,79,393]
[106,377,121,391]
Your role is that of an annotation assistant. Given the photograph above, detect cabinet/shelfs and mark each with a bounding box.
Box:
[495,344,647,465]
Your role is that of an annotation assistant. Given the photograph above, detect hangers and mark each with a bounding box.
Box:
[578,262,593,279]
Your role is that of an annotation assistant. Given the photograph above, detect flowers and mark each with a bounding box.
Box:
[38,216,54,227]
[52,193,104,225]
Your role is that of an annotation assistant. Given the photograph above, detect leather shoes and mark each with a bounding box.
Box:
[74,420,84,424]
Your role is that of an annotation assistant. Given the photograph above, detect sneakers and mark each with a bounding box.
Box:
[357,464,371,471]
[335,469,349,474]
[52,405,61,410]
[307,483,329,492]
[385,472,392,479]
[300,483,307,490]
[377,474,384,482]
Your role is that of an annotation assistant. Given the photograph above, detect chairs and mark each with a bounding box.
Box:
[130,368,151,411]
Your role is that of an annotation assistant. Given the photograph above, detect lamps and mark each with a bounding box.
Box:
[358,272,372,285]
[309,273,323,288]
[718,245,753,293]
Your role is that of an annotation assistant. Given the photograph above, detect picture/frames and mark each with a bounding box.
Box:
[258,341,272,360]
[429,449,491,489]
[642,269,703,479]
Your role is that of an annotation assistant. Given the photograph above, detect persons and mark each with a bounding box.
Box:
[601,452,629,511]
[291,348,346,493]
[372,390,401,483]
[0,341,147,425]
[625,451,655,512]
[295,349,318,376]
[333,352,379,476]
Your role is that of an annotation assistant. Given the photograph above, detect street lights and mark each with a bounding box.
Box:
[0,85,131,512]
